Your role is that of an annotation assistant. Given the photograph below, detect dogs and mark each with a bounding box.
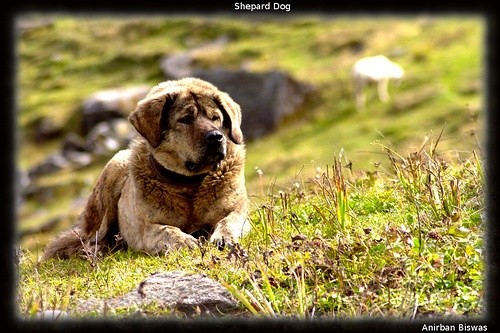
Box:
[40,77,252,262]
